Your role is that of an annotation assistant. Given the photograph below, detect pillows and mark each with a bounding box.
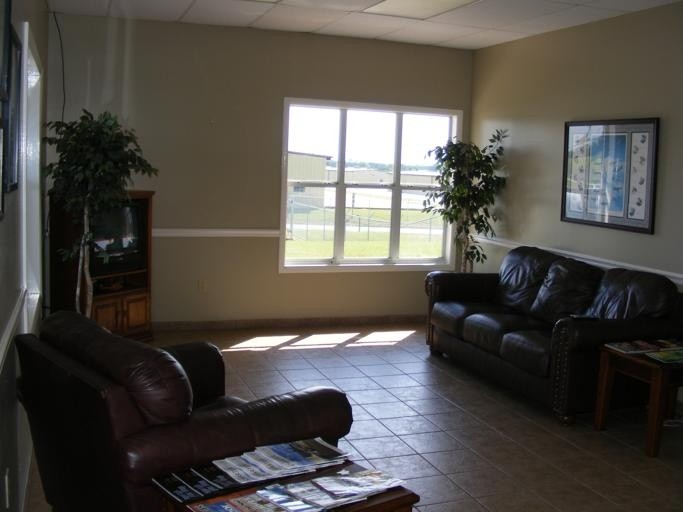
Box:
[39,306,194,426]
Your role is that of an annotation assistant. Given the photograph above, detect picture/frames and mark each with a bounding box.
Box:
[2,23,22,193]
[560,117,662,235]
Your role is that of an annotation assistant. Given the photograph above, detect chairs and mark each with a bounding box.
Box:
[15,310,353,512]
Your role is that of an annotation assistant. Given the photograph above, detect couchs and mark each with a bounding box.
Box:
[423,246,680,426]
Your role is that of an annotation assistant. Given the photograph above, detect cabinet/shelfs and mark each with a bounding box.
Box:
[46,268,156,344]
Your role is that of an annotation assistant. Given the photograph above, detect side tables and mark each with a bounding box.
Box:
[151,437,420,512]
[591,336,683,458]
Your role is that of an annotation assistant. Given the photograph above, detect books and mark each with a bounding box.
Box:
[151,435,408,512]
[603,336,683,363]
